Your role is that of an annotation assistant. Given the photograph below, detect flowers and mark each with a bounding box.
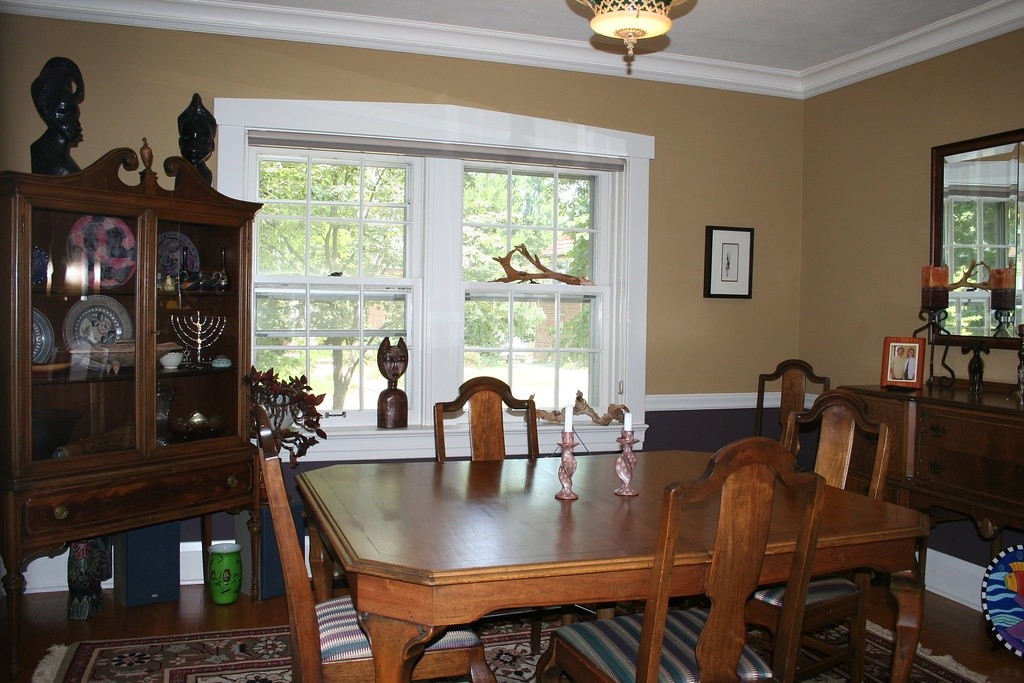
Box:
[240,365,328,470]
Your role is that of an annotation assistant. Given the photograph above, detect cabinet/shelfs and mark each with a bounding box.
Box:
[832,374,1024,563]
[0,136,260,604]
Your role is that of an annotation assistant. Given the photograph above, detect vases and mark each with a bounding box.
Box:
[204,541,243,607]
[259,396,300,429]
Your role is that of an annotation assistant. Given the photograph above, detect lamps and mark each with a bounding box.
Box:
[565,0,699,57]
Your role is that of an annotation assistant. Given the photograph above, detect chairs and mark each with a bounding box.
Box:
[433,375,544,654]
[248,401,498,683]
[742,388,897,683]
[754,358,832,436]
[633,433,828,683]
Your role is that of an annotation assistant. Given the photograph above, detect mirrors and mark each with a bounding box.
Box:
[928,126,1024,350]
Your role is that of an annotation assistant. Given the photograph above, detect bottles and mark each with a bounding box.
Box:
[156,246,232,293]
[207,543,243,605]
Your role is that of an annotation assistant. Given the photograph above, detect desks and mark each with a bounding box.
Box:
[292,448,931,683]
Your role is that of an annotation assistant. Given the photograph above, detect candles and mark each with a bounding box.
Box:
[988,267,1014,289]
[922,265,948,288]
[564,405,573,432]
[624,413,632,431]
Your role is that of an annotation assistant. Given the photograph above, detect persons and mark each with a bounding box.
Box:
[30,56,85,174]
[891,347,907,380]
[174,93,216,186]
[904,348,916,381]
[377,337,408,428]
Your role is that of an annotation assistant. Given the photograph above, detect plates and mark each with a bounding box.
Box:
[32,306,55,365]
[63,294,134,370]
[66,214,137,289]
[32,246,49,285]
[155,230,199,278]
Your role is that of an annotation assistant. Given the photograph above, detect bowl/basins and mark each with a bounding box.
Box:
[159,352,184,369]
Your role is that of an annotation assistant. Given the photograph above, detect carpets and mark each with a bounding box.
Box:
[34,590,986,683]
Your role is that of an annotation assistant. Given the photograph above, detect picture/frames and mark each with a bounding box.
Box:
[880,336,926,390]
[703,225,755,299]
